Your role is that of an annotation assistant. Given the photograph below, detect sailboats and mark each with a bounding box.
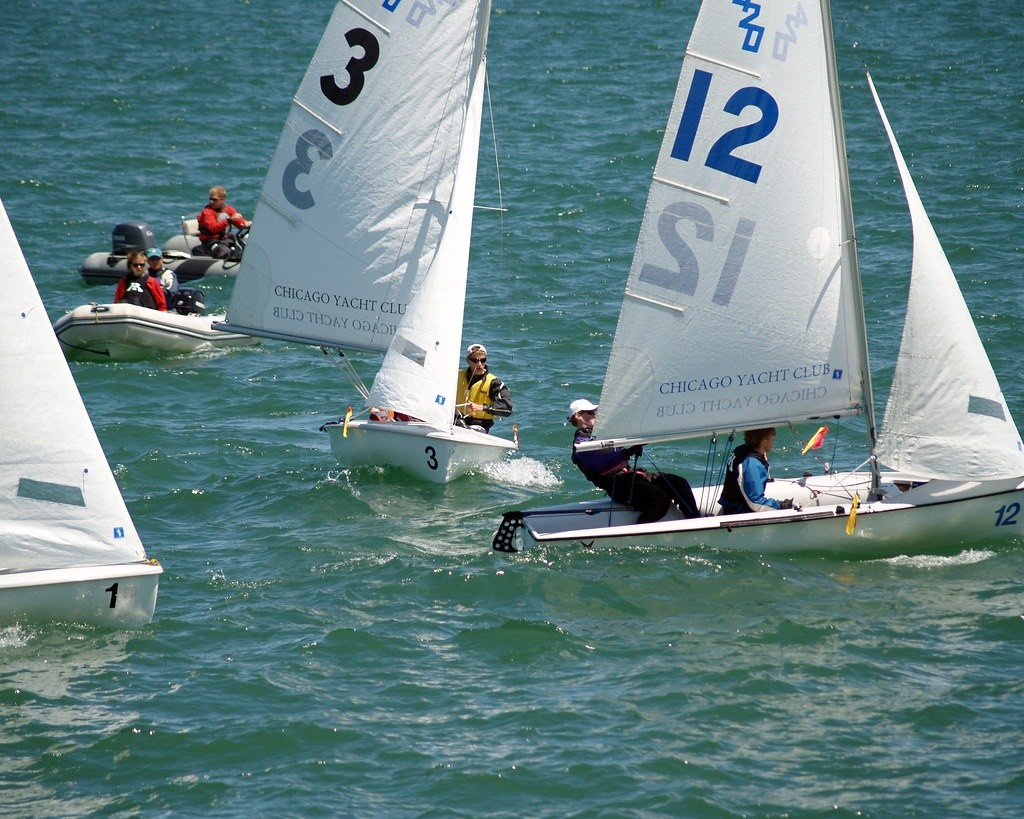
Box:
[208,1,522,487]
[0,198,164,634]
[494,0,1024,552]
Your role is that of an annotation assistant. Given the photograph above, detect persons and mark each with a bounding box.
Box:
[114,248,178,312]
[717,428,802,515]
[566,399,699,523]
[455,344,513,434]
[199,186,252,259]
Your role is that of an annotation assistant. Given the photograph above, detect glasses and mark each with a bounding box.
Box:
[209,197,221,201]
[468,357,486,363]
[131,262,146,268]
[582,410,594,415]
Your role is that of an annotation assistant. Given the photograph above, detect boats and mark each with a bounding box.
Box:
[75,214,249,282]
[53,300,263,363]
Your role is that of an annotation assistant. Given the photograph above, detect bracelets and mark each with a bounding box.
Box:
[482,405,487,410]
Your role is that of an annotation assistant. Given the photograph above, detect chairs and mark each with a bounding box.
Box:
[164,218,201,256]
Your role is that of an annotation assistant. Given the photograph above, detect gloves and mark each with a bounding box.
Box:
[629,445,643,457]
[781,499,793,509]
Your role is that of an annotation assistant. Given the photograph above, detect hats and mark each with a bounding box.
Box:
[146,248,162,258]
[467,344,487,358]
[569,399,599,419]
